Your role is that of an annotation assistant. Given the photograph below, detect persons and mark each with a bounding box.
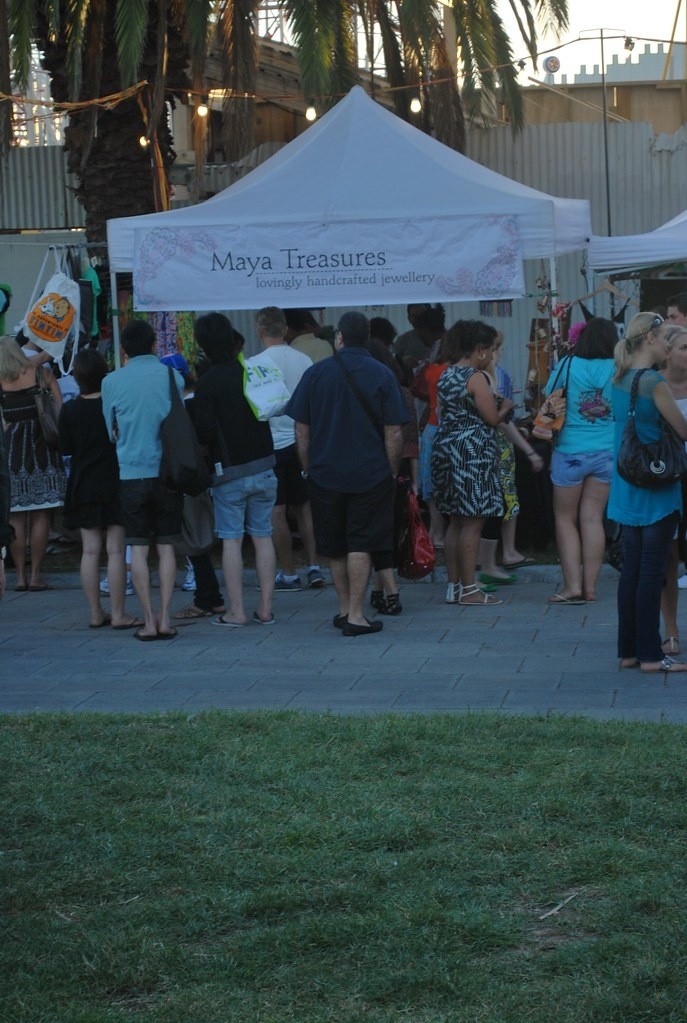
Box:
[0,303,542,642]
[543,292,687,673]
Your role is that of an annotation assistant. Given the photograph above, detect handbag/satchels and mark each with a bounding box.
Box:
[400,494,436,583]
[533,387,567,439]
[19,242,93,374]
[234,350,291,422]
[615,414,687,487]
[154,367,214,497]
[32,365,64,452]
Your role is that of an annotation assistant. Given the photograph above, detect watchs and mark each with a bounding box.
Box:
[301,470,308,477]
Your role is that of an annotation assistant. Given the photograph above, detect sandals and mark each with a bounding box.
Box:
[445,581,503,606]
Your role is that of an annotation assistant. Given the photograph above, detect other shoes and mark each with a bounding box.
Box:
[477,571,518,593]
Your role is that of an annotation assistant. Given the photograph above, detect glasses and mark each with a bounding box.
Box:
[651,313,664,331]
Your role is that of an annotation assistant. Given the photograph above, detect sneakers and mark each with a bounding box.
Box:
[305,565,327,586]
[182,556,197,592]
[369,590,402,613]
[98,571,135,596]
[256,571,303,592]
[334,613,383,636]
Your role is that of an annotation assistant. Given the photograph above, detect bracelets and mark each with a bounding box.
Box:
[526,451,537,456]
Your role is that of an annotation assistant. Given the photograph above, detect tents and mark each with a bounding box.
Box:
[106,85,590,370]
[588,209,687,315]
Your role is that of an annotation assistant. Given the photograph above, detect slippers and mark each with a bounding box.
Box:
[89,597,276,639]
[623,656,686,672]
[547,587,595,604]
[501,554,536,569]
[29,583,54,591]
[14,585,27,592]
[660,636,679,656]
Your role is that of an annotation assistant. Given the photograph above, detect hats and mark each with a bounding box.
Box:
[162,354,191,374]
